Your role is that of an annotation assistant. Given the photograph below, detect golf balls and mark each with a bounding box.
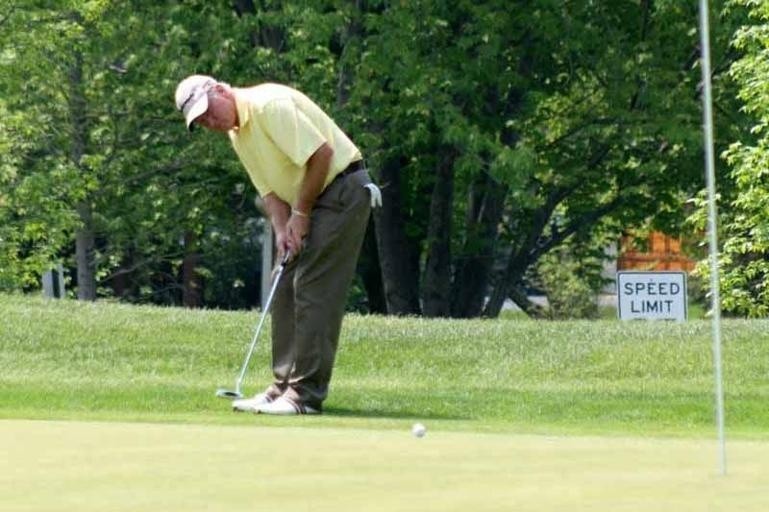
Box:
[413,424,425,436]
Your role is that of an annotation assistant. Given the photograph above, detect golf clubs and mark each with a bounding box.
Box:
[216,251,291,399]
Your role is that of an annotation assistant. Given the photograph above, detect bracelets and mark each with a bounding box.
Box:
[291,208,312,219]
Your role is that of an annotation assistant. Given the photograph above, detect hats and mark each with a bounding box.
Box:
[174,73,216,134]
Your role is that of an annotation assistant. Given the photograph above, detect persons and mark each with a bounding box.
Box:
[174,74,384,417]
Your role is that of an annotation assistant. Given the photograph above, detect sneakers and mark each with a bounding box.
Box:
[232,387,281,412]
[255,392,320,414]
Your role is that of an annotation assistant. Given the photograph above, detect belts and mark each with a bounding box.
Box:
[335,159,371,180]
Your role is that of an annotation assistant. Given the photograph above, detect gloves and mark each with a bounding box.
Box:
[364,183,384,208]
